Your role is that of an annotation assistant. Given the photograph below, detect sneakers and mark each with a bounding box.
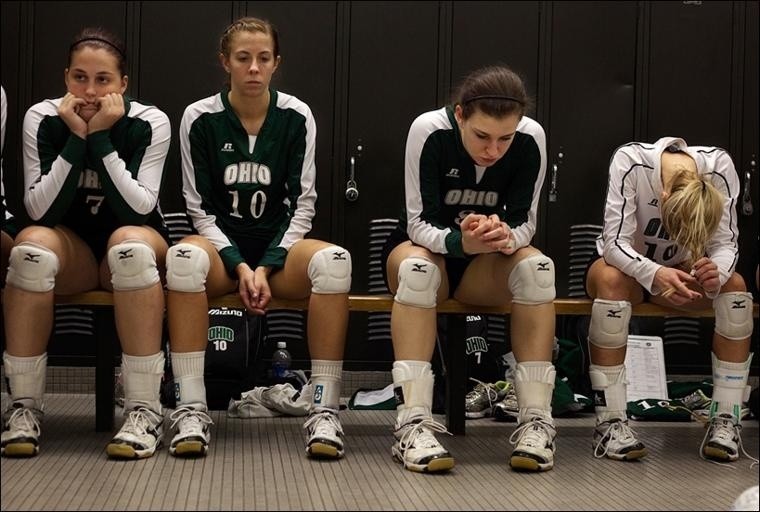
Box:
[509,363,557,472]
[589,365,649,461]
[304,373,344,460]
[391,361,456,473]
[465,377,521,425]
[700,352,752,461]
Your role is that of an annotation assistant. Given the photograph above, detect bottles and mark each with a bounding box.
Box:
[271,341,293,377]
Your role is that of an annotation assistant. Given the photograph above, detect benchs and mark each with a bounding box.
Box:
[0,289,758,435]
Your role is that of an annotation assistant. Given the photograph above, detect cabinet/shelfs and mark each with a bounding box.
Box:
[551,2,636,336]
[26,3,125,357]
[452,0,538,357]
[245,1,334,243]
[142,2,232,246]
[345,2,437,361]
[655,1,732,147]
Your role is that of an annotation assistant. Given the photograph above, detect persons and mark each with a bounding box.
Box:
[381,63,559,474]
[582,132,760,464]
[0,27,174,463]
[162,17,353,460]
[0,85,16,288]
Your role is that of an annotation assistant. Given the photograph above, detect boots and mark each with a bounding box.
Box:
[106,352,164,460]
[1,351,48,458]
[169,369,212,459]
